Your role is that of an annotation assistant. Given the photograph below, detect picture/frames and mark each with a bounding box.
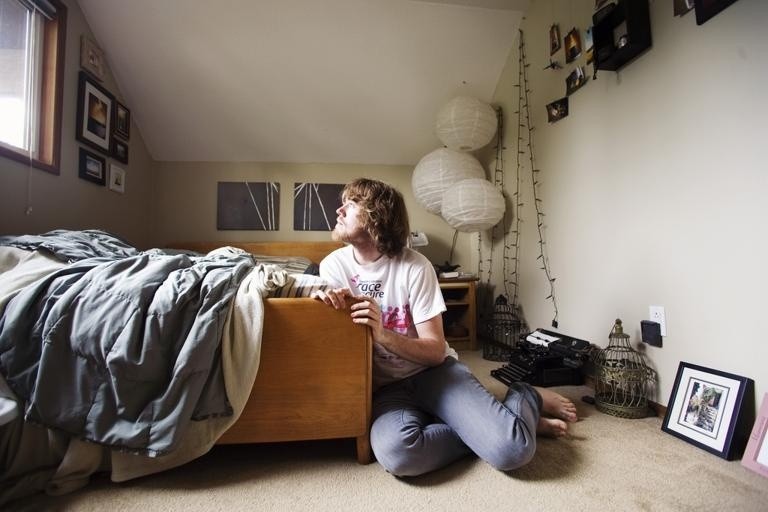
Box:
[110,162,125,194]
[112,137,130,166]
[79,146,106,187]
[661,362,754,460]
[294,182,346,232]
[217,181,280,231]
[739,392,767,479]
[79,33,106,82]
[114,100,131,142]
[75,71,116,157]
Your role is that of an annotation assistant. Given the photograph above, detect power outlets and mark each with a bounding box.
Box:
[650,305,666,336]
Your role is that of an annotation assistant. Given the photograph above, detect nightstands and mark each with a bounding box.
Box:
[436,278,480,351]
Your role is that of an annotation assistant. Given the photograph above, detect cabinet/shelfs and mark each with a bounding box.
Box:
[592,0,651,71]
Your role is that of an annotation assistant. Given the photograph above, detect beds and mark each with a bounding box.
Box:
[0,242,373,466]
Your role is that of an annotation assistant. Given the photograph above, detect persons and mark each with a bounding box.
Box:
[310,178,576,478]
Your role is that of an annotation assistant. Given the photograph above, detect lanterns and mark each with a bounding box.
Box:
[412,148,486,215]
[440,178,507,232]
[434,96,497,153]
